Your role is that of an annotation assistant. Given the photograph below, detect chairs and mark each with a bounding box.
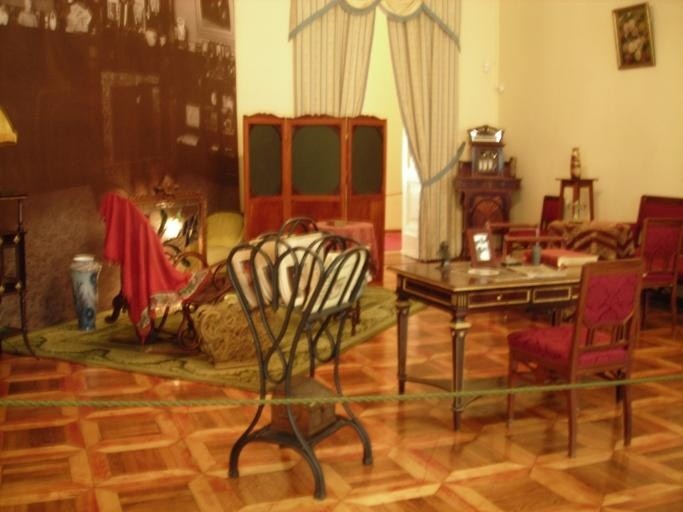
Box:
[634,193,682,221]
[96,186,237,349]
[486,194,566,258]
[503,259,648,460]
[635,217,682,330]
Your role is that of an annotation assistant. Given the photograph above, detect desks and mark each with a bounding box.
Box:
[546,217,638,262]
[556,176,598,224]
[385,259,624,431]
[308,220,380,274]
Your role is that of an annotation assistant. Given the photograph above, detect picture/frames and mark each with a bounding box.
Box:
[464,227,499,268]
[612,1,659,70]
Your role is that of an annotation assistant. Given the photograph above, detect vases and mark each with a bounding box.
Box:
[67,253,103,332]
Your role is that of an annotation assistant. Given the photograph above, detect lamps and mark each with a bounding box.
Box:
[0,108,17,144]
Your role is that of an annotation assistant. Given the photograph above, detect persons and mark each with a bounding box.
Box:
[17,0,37,29]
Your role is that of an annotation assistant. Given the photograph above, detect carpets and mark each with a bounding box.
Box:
[0,284,428,394]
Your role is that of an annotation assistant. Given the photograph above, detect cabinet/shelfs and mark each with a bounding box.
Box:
[0,190,43,364]
[219,216,376,501]
[455,156,522,260]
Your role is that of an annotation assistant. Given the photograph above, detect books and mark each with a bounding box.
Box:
[523,248,600,269]
[231,230,373,314]
